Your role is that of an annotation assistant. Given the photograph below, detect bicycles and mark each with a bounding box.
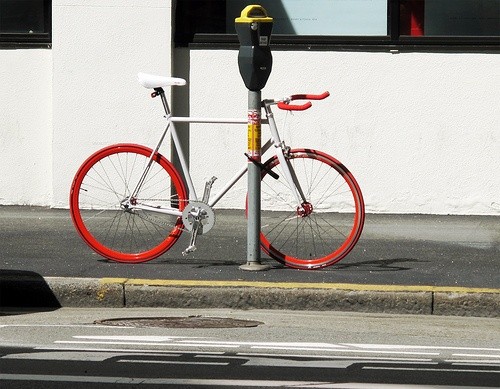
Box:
[69,72,365,270]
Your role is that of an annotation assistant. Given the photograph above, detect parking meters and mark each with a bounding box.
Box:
[234,3,273,271]
